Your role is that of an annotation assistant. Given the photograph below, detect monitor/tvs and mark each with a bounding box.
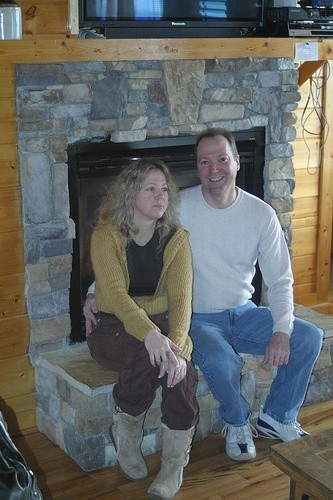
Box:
[77,0,264,38]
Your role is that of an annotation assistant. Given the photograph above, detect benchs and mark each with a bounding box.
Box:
[35,306,333,472]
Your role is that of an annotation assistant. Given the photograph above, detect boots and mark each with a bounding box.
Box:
[109,403,148,481]
[146,422,195,500]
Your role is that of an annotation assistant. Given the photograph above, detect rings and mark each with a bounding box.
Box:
[176,371,180,375]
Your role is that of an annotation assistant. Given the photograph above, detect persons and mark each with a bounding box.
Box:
[86,158,200,500]
[83,128,323,461]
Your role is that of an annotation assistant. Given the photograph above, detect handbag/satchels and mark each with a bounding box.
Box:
[0,412,42,500]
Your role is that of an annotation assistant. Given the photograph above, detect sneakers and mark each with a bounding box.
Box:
[254,405,310,442]
[221,421,258,461]
